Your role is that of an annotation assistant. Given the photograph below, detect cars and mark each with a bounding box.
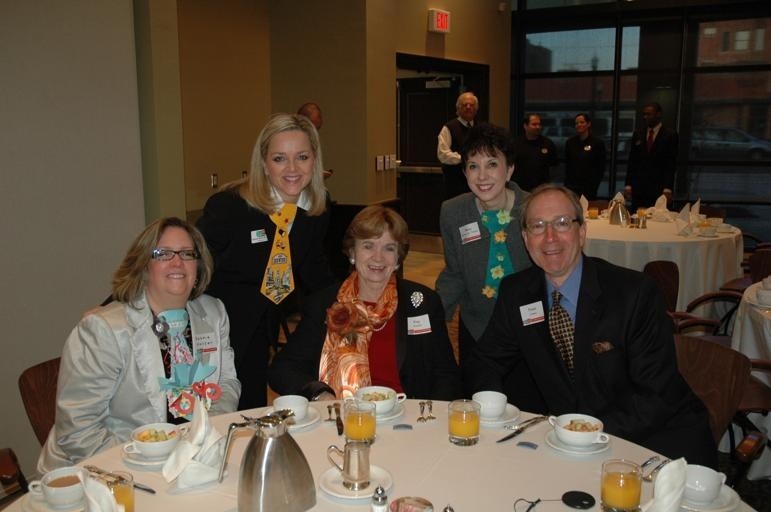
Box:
[691,124,770,164]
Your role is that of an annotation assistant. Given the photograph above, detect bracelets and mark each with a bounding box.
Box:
[311,389,328,401]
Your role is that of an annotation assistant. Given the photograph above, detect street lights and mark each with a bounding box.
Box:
[589,52,599,108]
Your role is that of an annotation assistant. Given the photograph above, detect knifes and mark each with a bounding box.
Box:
[85,464,158,495]
[496,416,547,444]
[333,402,344,436]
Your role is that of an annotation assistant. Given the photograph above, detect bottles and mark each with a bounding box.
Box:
[417,400,438,423]
[444,504,455,512]
[370,485,390,512]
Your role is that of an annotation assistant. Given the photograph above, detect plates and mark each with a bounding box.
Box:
[546,428,610,454]
[261,406,320,429]
[679,484,740,512]
[319,463,392,499]
[122,451,169,466]
[22,485,87,512]
[479,404,521,426]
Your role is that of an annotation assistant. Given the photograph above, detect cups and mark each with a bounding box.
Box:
[389,496,435,512]
[588,206,737,237]
[473,391,509,419]
[274,394,310,421]
[344,386,407,414]
[602,459,643,512]
[681,462,727,506]
[344,400,377,444]
[97,470,137,512]
[447,398,481,445]
[376,403,405,421]
[28,467,90,508]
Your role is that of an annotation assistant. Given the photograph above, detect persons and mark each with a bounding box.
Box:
[623,101,679,217]
[509,112,556,196]
[430,127,536,375]
[561,112,606,201]
[462,182,719,471]
[36,217,240,480]
[436,91,480,203]
[297,102,333,182]
[192,113,348,412]
[268,204,460,404]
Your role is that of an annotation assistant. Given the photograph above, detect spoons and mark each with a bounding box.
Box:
[325,404,336,422]
[643,458,671,482]
[504,415,543,430]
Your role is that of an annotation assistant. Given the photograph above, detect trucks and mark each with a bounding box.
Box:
[535,112,635,165]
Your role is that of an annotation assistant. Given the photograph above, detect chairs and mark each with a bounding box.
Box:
[644,242,771,488]
[19,356,60,448]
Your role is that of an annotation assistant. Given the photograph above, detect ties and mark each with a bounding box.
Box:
[647,129,654,151]
[548,292,575,376]
[261,203,299,304]
[481,209,516,301]
[157,309,212,421]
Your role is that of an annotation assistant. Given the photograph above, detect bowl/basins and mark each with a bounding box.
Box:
[124,423,188,459]
[549,412,610,445]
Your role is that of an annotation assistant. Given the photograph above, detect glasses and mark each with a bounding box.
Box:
[151,248,197,261]
[523,216,579,235]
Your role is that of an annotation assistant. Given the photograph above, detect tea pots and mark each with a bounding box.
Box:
[607,199,631,224]
[219,410,318,512]
[328,440,374,489]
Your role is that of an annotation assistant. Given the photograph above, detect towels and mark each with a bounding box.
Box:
[578,192,702,237]
[77,470,125,512]
[649,455,688,509]
[162,395,227,487]
